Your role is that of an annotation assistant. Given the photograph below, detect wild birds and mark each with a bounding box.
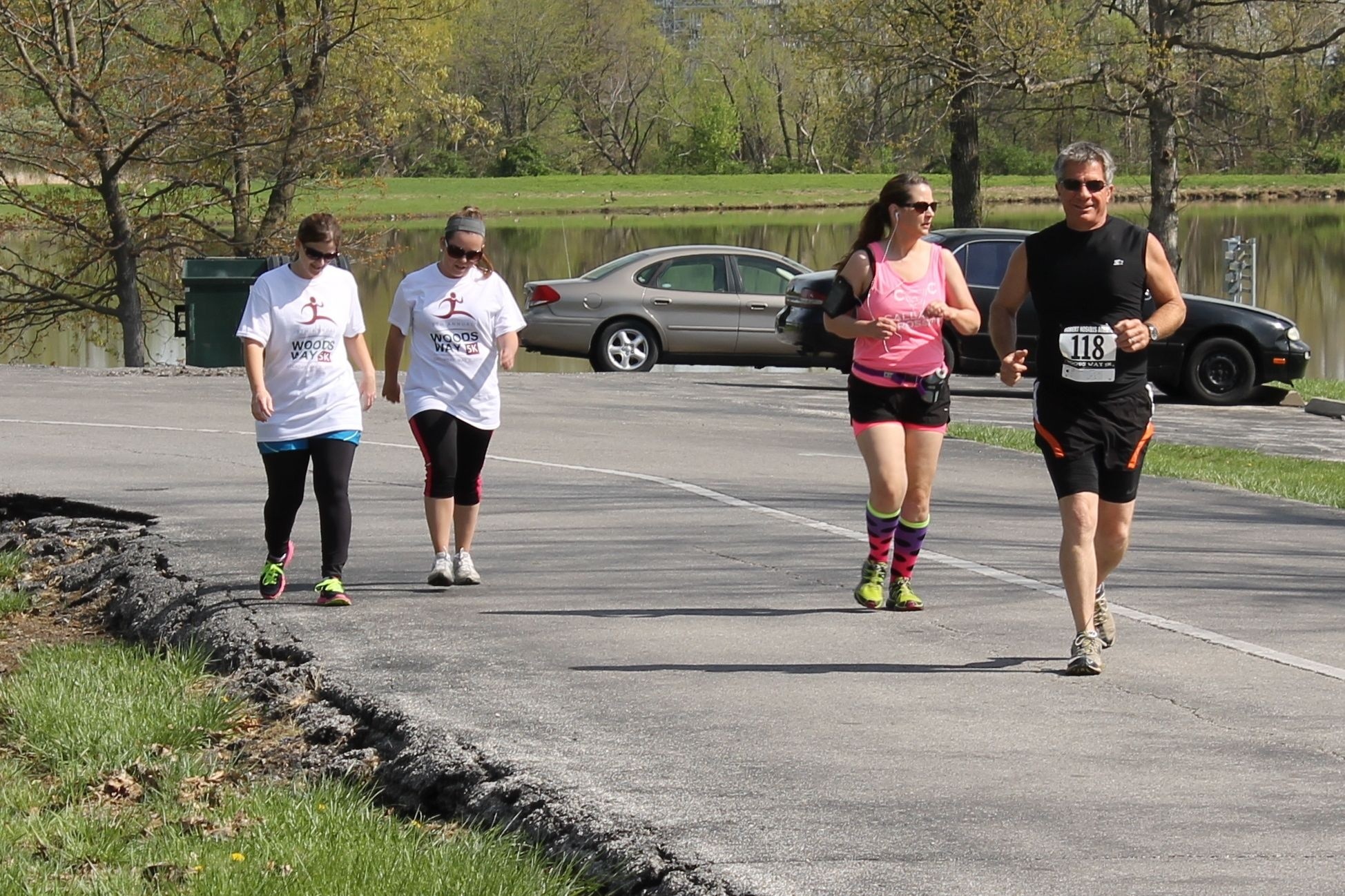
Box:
[601,190,618,206]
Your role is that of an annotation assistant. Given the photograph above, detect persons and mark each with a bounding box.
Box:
[988,140,1187,675]
[823,171,981,610]
[379,205,527,589]
[235,214,376,609]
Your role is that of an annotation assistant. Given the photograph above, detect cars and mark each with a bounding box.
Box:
[773,228,1312,406]
[515,245,821,374]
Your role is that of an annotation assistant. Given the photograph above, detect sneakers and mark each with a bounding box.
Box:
[1068,628,1107,676]
[454,548,481,585]
[258,540,294,599]
[427,552,454,586]
[1093,582,1116,647]
[314,576,351,606]
[854,558,888,609]
[886,577,926,610]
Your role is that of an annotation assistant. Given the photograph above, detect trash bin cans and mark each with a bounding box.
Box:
[266,252,352,271]
[174,256,266,367]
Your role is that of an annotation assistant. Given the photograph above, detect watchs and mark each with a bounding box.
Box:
[1144,322,1162,344]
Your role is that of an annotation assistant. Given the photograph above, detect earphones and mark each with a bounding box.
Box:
[895,211,900,222]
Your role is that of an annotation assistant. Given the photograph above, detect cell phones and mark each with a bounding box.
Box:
[822,276,851,318]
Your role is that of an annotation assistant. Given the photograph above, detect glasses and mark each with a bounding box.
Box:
[1058,177,1107,191]
[897,201,939,214]
[299,240,339,261]
[443,238,483,261]
